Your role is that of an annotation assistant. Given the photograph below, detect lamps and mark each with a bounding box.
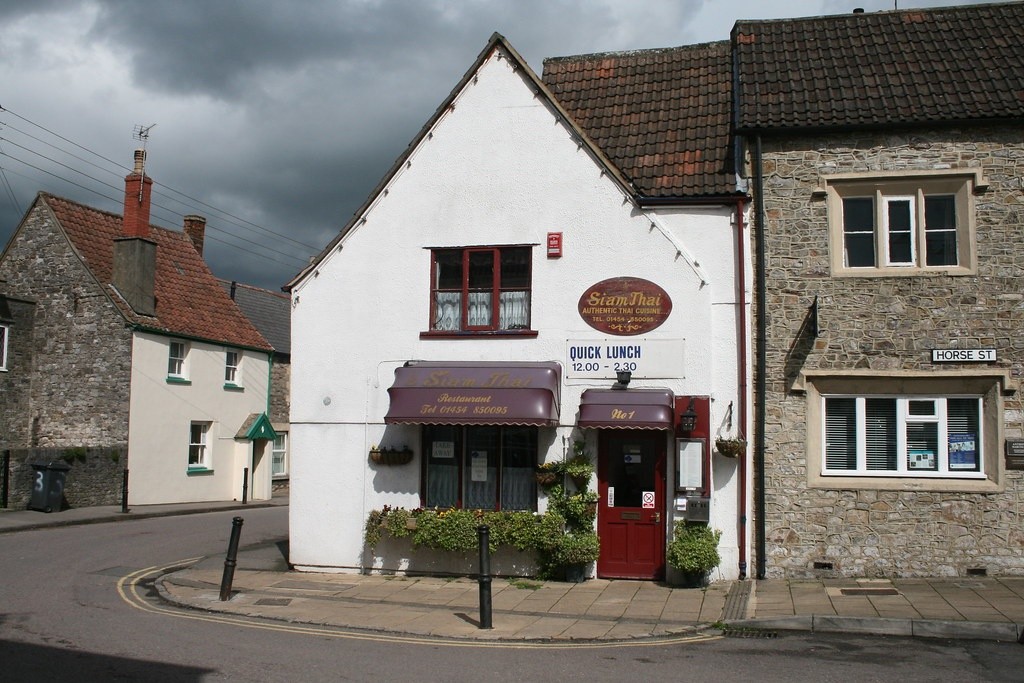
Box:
[616,369,632,387]
[680,398,698,431]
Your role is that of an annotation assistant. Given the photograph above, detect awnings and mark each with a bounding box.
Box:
[382,361,563,427]
[575,388,676,431]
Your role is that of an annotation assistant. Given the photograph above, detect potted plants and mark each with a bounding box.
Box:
[560,532,601,583]
[564,441,599,491]
[568,489,599,519]
[663,519,723,587]
[370,444,413,465]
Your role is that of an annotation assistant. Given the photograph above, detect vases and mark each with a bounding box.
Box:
[380,516,388,528]
[537,472,556,485]
[406,517,418,530]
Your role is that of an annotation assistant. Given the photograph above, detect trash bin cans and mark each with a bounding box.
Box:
[26,461,71,513]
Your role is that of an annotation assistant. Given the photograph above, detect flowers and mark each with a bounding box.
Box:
[537,460,565,473]
[380,505,392,517]
[411,506,423,517]
